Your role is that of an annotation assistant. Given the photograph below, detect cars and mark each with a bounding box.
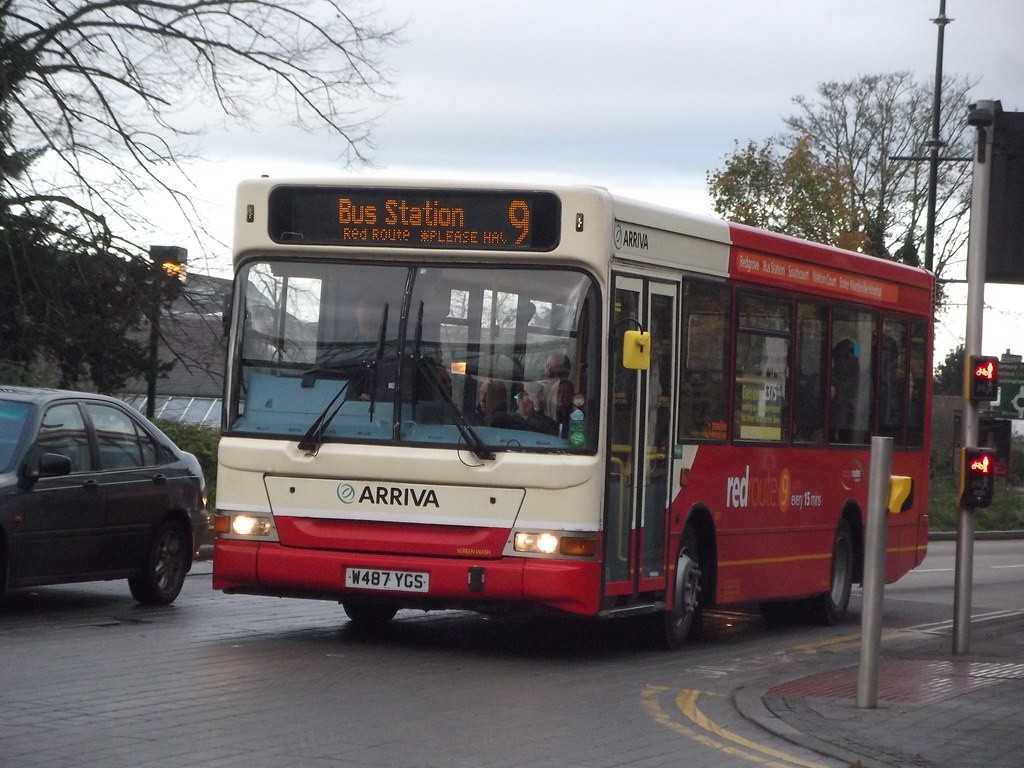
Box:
[0,382,208,605]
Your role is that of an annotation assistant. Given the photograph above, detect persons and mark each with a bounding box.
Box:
[534,353,573,435]
[828,333,901,443]
[512,386,559,437]
[646,315,672,445]
[466,378,531,431]
[316,296,418,403]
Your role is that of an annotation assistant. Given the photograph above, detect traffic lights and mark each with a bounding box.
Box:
[963,444,995,507]
[969,356,999,402]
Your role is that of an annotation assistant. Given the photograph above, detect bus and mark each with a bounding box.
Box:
[216,169,938,649]
